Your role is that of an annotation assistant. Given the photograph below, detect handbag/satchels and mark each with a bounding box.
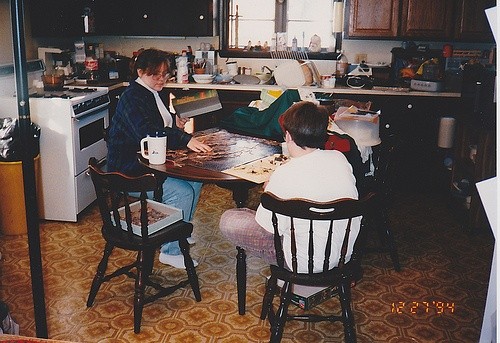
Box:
[0,117,40,161]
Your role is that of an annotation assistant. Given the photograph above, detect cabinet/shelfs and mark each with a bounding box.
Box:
[451,0,496,44]
[95,0,214,36]
[28,0,95,40]
[343,0,453,42]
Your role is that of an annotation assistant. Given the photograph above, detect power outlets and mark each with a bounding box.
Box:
[356,54,367,64]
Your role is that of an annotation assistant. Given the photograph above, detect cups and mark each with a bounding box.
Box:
[140,132,167,165]
[442,45,453,58]
[320,75,336,89]
[239,67,245,75]
[245,68,252,75]
[195,69,206,74]
[225,61,238,76]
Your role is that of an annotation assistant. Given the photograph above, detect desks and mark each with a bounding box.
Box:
[138,128,281,316]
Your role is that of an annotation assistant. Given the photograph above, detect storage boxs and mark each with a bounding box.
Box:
[110,198,183,237]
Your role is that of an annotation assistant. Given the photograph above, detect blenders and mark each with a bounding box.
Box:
[38,46,74,84]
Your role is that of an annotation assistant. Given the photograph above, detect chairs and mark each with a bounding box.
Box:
[86,157,202,335]
[260,191,378,343]
[364,130,401,272]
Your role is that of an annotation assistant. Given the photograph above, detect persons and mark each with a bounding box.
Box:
[219,99,363,272]
[109,48,214,269]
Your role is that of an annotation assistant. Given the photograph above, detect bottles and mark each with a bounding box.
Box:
[309,34,322,52]
[336,50,348,84]
[130,48,144,79]
[85,43,120,82]
[292,37,298,51]
[402,40,407,49]
[200,42,210,51]
[172,45,194,84]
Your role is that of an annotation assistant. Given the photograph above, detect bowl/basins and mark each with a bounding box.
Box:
[192,74,214,83]
[334,107,382,146]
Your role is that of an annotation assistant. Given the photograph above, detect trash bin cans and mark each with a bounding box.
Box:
[0,154,41,237]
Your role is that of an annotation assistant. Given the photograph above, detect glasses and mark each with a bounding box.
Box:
[152,72,170,79]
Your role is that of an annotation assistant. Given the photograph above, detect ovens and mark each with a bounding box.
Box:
[0,100,111,223]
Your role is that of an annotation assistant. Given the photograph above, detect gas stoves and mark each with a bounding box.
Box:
[0,58,110,118]
